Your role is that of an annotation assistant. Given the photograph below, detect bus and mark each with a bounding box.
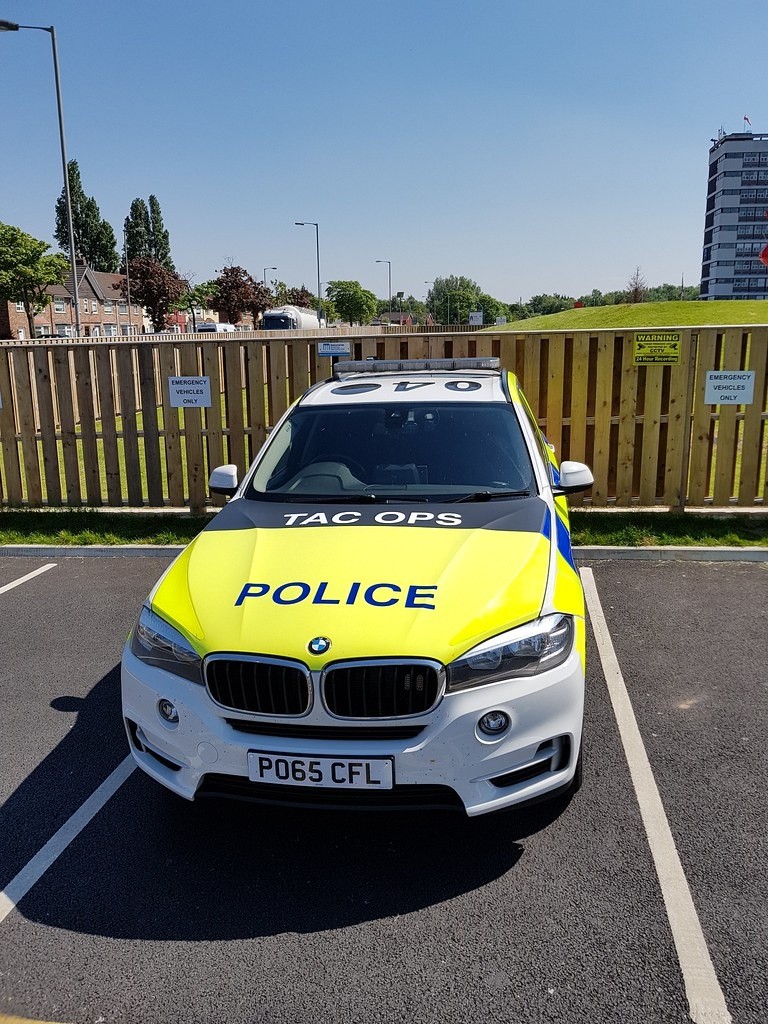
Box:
[197,322,234,333]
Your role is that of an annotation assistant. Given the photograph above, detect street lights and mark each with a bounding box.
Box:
[122,228,132,334]
[295,221,322,328]
[375,260,392,328]
[264,268,278,288]
[425,281,436,332]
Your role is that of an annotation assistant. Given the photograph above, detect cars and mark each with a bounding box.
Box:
[118,357,594,829]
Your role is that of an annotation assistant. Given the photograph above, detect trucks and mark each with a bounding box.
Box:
[261,305,326,329]
